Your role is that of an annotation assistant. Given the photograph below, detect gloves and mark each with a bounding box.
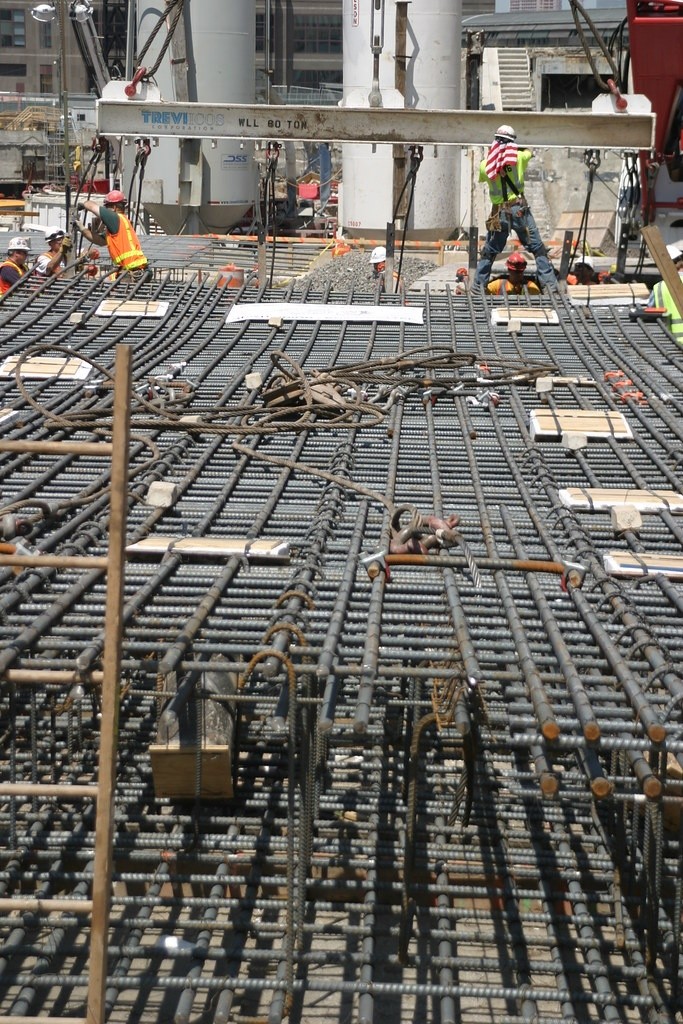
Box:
[59,235,72,252]
[77,198,87,210]
[75,219,85,232]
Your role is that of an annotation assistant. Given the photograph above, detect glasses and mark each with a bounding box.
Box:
[576,265,582,268]
[50,230,64,239]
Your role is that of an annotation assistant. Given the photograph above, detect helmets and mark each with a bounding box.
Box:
[45,227,65,242]
[494,125,517,142]
[575,256,594,273]
[505,253,527,271]
[102,190,127,205]
[609,265,616,274]
[369,246,386,263]
[666,245,682,259]
[8,237,31,250]
[456,268,468,276]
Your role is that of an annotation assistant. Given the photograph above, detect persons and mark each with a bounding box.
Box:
[456,254,623,297]
[369,246,407,304]
[646,244,683,346]
[0,191,153,302]
[469,125,563,296]
